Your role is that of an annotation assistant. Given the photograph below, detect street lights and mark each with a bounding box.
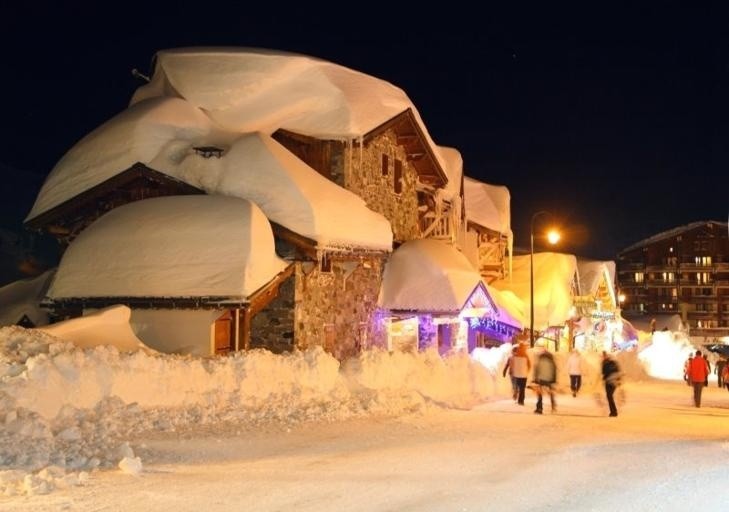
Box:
[528,209,566,346]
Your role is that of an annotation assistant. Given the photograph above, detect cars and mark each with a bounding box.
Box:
[702,344,729,354]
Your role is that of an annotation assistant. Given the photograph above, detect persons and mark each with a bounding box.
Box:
[510,343,531,405]
[683,350,729,407]
[566,348,585,397]
[601,351,622,418]
[534,350,556,414]
[502,348,517,398]
[650,317,656,334]
